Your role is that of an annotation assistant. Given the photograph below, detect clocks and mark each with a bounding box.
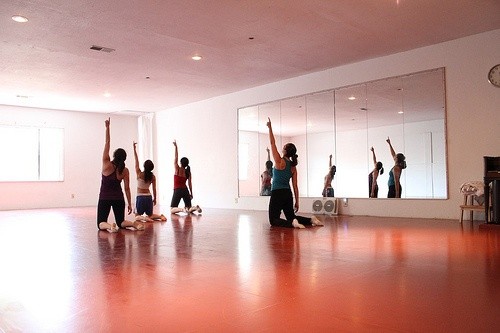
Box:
[488,64,500,88]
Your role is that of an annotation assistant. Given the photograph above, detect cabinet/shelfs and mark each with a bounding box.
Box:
[483,156,500,224]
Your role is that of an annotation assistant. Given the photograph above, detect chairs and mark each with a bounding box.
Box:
[460,191,492,223]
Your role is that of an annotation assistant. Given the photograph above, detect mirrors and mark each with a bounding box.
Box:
[236,66,448,200]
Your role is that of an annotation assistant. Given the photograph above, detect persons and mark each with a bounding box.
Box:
[321,154,336,197]
[260,147,274,196]
[169,139,202,214]
[386,137,408,198]
[132,140,167,221]
[266,117,325,229]
[96,117,145,233]
[368,146,384,198]
